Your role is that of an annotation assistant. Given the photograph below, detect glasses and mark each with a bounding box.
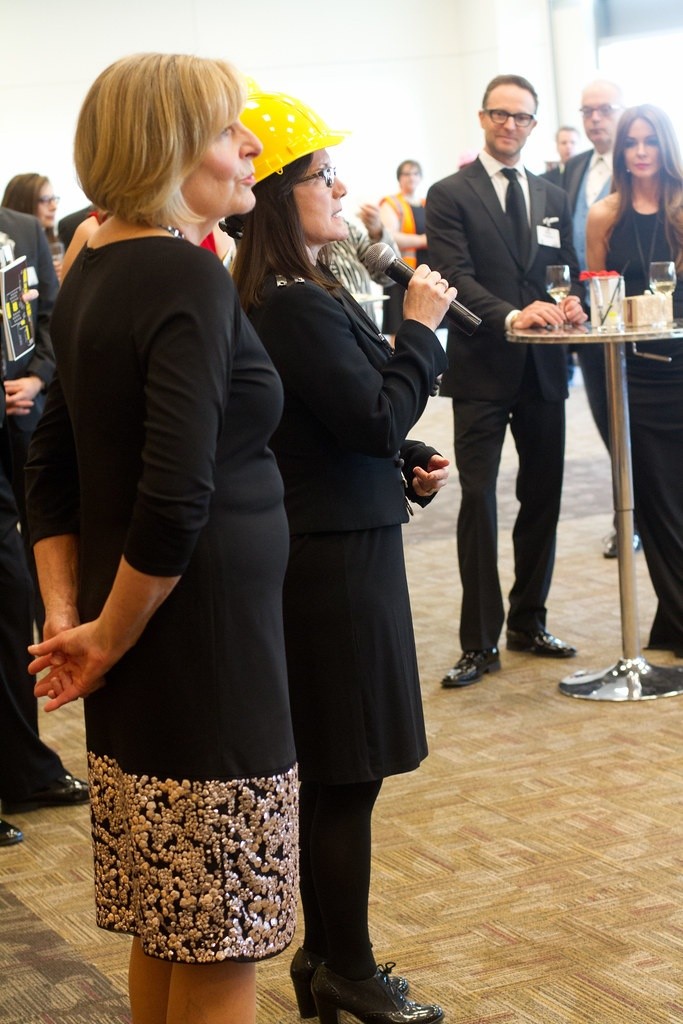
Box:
[279,166,336,197]
[38,196,60,205]
[580,105,621,117]
[485,109,537,128]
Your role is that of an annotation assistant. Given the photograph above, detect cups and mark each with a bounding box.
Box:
[590,273,626,334]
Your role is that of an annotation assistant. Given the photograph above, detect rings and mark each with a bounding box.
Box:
[436,281,448,289]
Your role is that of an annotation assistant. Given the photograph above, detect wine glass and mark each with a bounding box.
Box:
[648,261,678,329]
[545,264,573,330]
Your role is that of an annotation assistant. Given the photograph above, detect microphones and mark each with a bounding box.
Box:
[364,242,483,335]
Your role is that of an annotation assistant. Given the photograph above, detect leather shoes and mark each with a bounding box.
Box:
[603,528,642,558]
[2,772,91,814]
[507,627,575,656]
[442,646,501,685]
[0,819,22,845]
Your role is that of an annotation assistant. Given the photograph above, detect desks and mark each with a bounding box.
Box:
[506,320,683,701]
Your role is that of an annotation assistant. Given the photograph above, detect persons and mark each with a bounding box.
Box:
[561,78,641,557]
[537,127,580,189]
[0,178,246,845]
[22,54,300,1024]
[375,159,431,345]
[586,104,683,659]
[317,203,403,332]
[423,74,579,689]
[222,88,460,1024]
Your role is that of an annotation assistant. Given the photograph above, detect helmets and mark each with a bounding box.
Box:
[238,93,353,188]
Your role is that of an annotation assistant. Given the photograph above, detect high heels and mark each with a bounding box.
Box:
[311,963,444,1024]
[290,947,409,1018]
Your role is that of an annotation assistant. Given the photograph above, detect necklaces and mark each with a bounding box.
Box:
[158,223,184,239]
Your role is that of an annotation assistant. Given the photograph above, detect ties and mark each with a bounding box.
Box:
[501,168,531,270]
